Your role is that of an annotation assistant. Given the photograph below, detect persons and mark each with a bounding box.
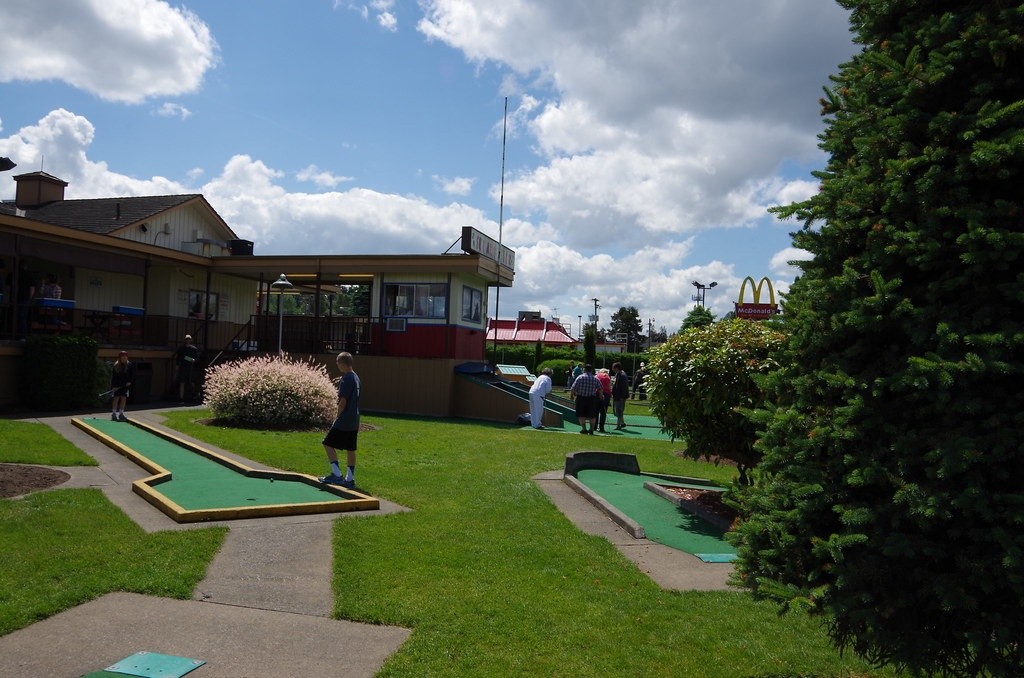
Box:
[176,334,198,406]
[528,367,552,429]
[631,367,648,399]
[5,256,66,344]
[321,352,361,490]
[565,360,583,389]
[612,362,630,430]
[569,363,611,435]
[111,350,134,421]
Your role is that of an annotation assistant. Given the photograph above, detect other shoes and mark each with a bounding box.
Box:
[318,473,344,485]
[614,426,621,430]
[620,423,626,428]
[111,413,117,421]
[579,429,588,434]
[587,429,593,434]
[533,425,545,429]
[119,413,127,419]
[192,398,197,404]
[338,479,355,488]
[173,400,185,405]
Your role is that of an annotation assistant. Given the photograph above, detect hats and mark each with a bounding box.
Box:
[185,334,192,340]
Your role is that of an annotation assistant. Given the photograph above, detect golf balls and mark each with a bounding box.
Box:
[271,477,273,483]
[94,417,96,419]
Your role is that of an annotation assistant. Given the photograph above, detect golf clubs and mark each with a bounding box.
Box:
[602,399,612,434]
[97,381,138,397]
[545,394,549,428]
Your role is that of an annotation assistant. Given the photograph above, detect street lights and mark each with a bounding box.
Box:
[578,315,582,342]
[691,281,718,308]
[271,274,293,352]
